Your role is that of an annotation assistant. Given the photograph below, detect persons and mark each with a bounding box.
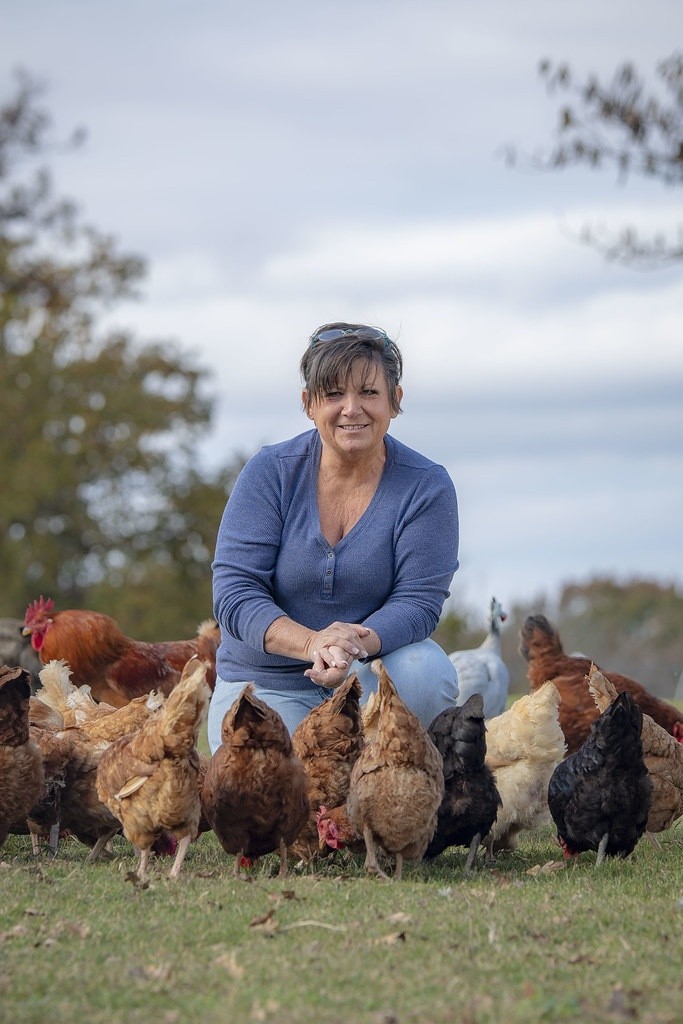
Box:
[208,321,461,759]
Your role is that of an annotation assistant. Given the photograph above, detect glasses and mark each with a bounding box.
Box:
[313,326,401,383]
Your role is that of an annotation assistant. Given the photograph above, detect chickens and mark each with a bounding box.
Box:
[0,594,683,888]
